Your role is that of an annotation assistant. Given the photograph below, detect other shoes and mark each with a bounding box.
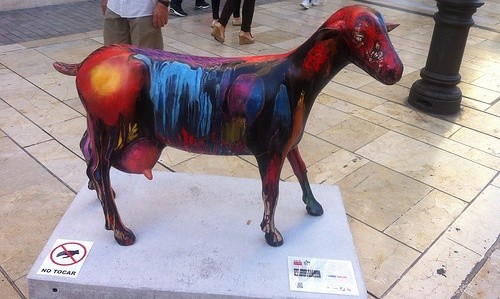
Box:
[211,19,219,28]
[170,2,187,17]
[232,17,242,26]
[312,0,326,6]
[195,2,210,9]
[300,0,313,9]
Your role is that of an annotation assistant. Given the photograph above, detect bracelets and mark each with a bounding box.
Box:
[158,0,170,8]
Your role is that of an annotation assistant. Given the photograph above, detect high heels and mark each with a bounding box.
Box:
[239,32,255,45]
[211,25,226,43]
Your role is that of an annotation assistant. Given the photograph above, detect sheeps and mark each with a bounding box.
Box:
[51,4,407,248]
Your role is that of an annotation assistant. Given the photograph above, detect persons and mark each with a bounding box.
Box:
[170,1,256,45]
[299,0,326,9]
[101,0,168,52]
